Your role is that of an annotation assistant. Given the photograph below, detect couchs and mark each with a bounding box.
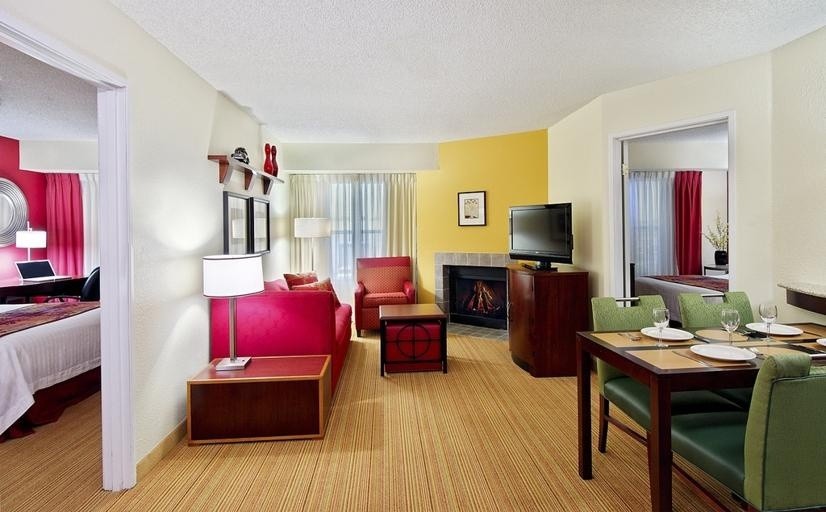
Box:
[210,280,352,401]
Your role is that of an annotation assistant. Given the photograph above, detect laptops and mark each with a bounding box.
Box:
[14,258,72,283]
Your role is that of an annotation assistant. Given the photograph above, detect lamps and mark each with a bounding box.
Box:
[14,221,48,261]
[294,217,333,272]
[201,254,266,373]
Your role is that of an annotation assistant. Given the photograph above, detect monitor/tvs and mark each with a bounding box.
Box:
[508,203,573,272]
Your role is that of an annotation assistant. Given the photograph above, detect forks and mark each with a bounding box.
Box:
[617,332,639,342]
[626,330,643,339]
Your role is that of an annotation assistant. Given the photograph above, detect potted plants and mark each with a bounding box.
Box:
[699,213,728,265]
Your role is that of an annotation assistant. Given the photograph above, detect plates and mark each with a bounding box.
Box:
[745,322,804,336]
[690,345,757,361]
[817,338,826,347]
[640,327,695,340]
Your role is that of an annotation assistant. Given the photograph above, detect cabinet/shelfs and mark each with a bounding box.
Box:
[504,263,589,378]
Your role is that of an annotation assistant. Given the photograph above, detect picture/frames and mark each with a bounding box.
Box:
[222,190,273,257]
[457,190,486,226]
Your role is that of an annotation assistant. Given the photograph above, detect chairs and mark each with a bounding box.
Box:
[354,255,417,337]
[81,267,99,301]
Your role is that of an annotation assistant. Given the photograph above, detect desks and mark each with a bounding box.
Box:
[0,275,89,302]
[185,354,334,447]
[703,264,728,275]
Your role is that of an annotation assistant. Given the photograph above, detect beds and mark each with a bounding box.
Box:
[0,303,101,444]
[635,274,728,327]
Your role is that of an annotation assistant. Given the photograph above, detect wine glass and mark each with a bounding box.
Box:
[653,308,671,348]
[721,309,741,345]
[759,303,778,341]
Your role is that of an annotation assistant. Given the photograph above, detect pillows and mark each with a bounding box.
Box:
[283,271,341,309]
